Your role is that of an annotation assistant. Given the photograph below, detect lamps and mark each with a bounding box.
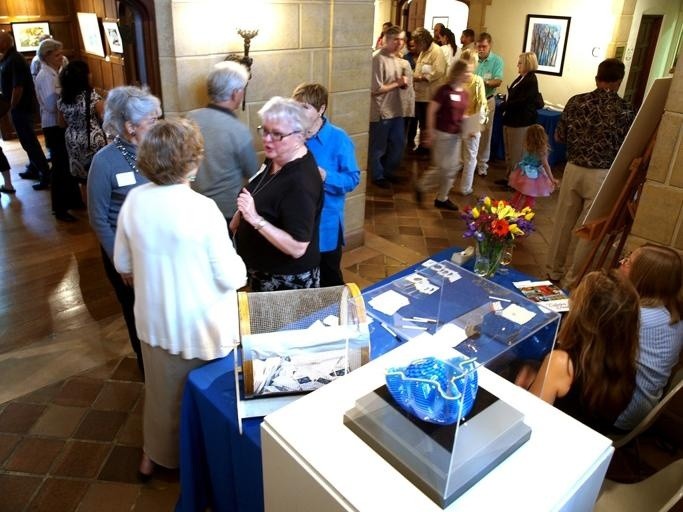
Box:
[224,2,265,111]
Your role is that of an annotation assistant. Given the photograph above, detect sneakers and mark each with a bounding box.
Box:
[478,163,487,175]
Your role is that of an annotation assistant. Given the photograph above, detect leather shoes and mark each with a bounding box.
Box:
[19,171,84,222]
[435,199,458,211]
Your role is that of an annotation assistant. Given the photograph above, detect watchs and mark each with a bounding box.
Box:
[254,216,266,232]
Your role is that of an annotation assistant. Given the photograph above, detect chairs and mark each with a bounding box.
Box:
[594,458,683,512]
[609,367,683,447]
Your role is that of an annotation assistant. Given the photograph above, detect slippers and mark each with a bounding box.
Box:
[0,185,16,193]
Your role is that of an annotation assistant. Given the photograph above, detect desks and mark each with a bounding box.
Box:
[489,96,563,166]
[176,244,579,512]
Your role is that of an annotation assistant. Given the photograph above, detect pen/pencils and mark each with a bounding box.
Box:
[402,316,439,331]
[489,296,512,303]
[380,322,402,343]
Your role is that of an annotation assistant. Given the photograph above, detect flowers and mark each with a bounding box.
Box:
[454,187,547,247]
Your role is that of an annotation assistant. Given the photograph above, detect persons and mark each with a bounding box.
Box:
[292,81,366,289]
[179,61,264,238]
[548,54,635,285]
[611,244,681,428]
[90,85,166,384]
[372,21,557,210]
[516,269,641,428]
[113,119,249,480]
[229,97,329,334]
[1,29,104,224]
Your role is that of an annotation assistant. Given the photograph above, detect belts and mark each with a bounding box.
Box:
[487,95,493,99]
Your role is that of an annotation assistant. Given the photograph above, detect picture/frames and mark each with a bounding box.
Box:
[101,17,123,58]
[431,16,449,31]
[11,20,53,54]
[522,14,572,76]
[76,11,106,61]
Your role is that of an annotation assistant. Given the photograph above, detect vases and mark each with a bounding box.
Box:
[471,233,515,277]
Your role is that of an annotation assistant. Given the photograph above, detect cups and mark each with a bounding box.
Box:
[496,246,513,275]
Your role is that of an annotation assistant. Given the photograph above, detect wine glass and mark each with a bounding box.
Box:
[463,313,483,353]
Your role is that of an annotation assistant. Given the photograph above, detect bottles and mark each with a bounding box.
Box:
[400,66,408,90]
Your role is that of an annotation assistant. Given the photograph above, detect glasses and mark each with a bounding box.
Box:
[257,126,300,141]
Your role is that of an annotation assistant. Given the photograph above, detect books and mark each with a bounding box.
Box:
[512,278,570,313]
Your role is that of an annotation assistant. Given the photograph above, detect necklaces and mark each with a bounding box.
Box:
[251,163,280,199]
